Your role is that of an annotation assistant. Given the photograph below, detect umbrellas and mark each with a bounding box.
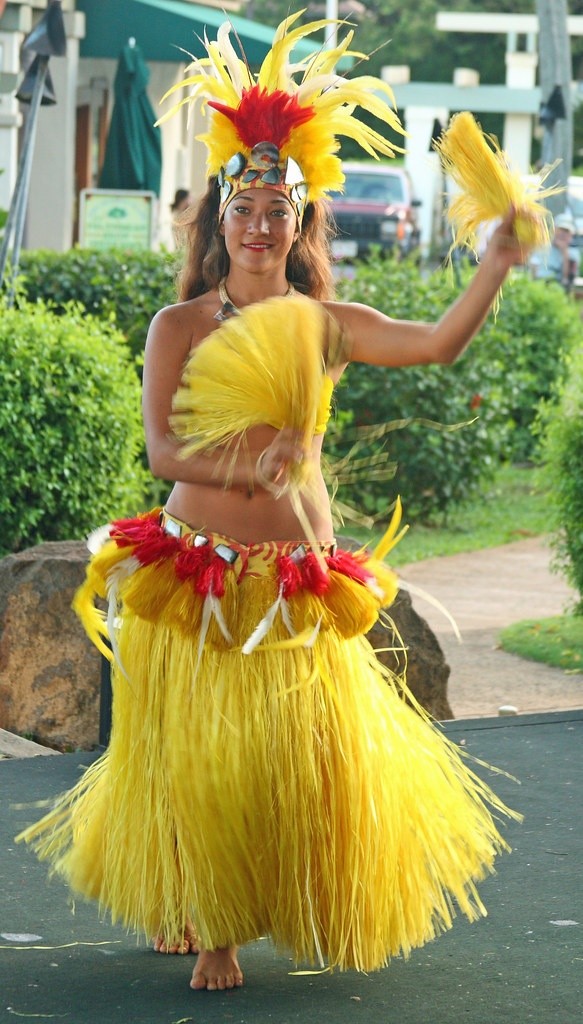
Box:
[98,45,162,199]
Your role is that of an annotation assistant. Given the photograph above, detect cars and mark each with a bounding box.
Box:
[313,161,424,266]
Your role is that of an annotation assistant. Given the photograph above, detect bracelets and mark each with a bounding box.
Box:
[251,445,291,501]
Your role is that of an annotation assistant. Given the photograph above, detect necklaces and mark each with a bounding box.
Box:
[214,276,296,322]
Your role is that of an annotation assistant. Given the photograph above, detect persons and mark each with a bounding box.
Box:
[540,214,577,299]
[143,144,537,991]
[170,189,190,254]
